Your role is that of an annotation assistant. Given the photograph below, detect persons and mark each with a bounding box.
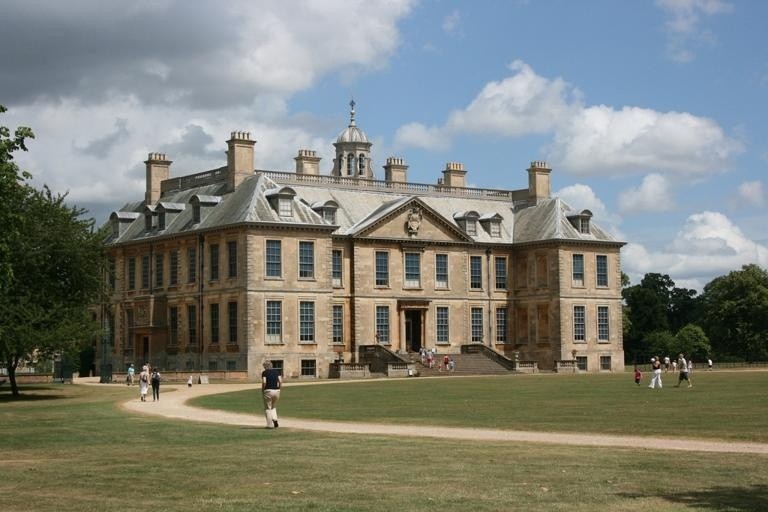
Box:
[143,361,152,385]
[261,361,282,430]
[139,365,150,402]
[149,368,161,401]
[633,354,714,389]
[418,346,456,376]
[127,363,135,386]
